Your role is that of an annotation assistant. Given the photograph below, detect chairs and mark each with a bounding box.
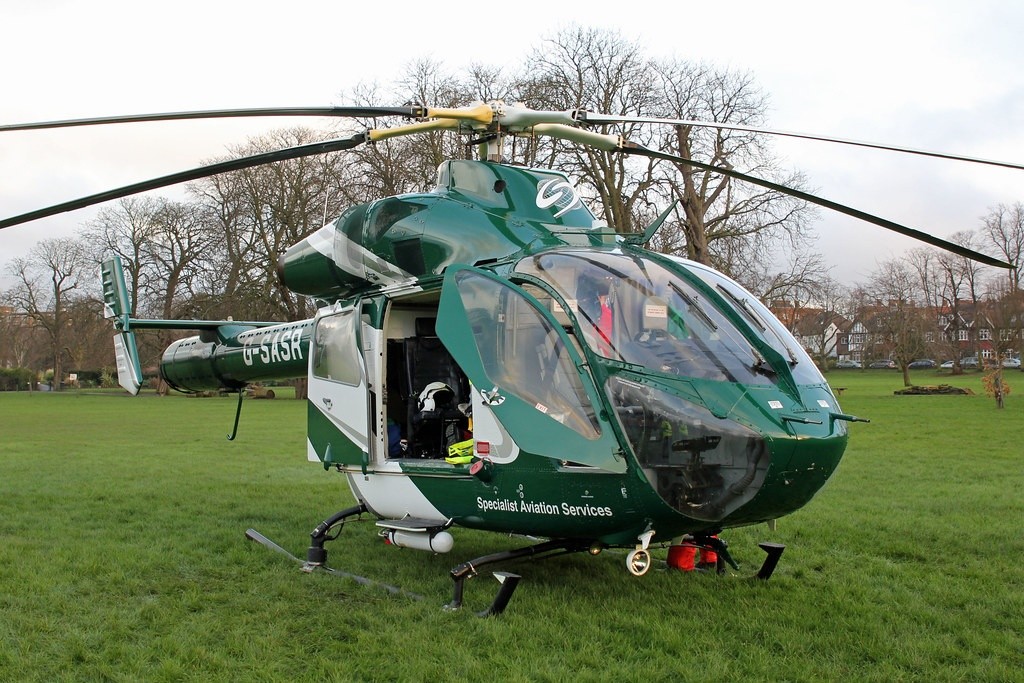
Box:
[535,327,600,430]
[404,334,472,458]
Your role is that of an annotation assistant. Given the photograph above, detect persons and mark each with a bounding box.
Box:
[677,420,688,439]
[661,417,672,458]
[666,535,717,570]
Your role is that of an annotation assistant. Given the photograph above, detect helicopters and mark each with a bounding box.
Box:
[1,101,1024,619]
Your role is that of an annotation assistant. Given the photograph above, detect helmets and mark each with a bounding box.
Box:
[416,381,456,410]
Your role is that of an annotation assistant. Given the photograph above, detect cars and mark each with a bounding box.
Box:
[960,357,979,368]
[870,360,896,368]
[908,360,937,368]
[952,360,963,367]
[1014,353,1020,360]
[836,360,862,368]
[941,361,954,368]
[1002,359,1021,368]
